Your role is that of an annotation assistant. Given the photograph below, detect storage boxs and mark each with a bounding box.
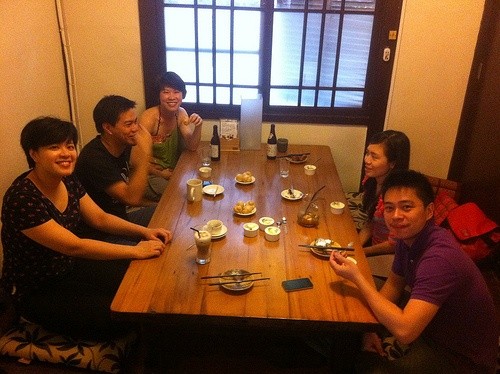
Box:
[219,133,240,151]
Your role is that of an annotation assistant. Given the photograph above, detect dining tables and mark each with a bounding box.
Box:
[109,141,381,374]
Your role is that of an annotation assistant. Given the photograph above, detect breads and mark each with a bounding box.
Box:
[233,200,256,214]
[183,116,191,126]
[236,171,252,182]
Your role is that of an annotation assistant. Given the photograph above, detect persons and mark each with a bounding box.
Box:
[0,71,203,341]
[329,129,500,374]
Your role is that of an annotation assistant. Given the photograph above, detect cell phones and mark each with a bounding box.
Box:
[282,278,313,292]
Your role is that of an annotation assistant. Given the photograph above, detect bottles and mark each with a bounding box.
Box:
[210,125,220,161]
[266,123,277,160]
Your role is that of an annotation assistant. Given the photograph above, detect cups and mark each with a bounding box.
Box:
[346,256,357,264]
[207,220,223,235]
[297,193,319,227]
[186,179,202,202]
[277,137,288,156]
[194,230,212,264]
[199,167,212,178]
[202,147,212,166]
[329,201,345,215]
[280,157,290,177]
[242,217,281,242]
[304,164,317,175]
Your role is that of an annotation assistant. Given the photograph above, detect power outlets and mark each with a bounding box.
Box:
[383,48,390,62]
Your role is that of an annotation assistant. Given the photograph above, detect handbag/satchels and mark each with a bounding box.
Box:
[436,200,500,265]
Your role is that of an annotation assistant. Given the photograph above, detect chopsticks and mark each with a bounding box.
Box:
[298,244,354,251]
[200,271,271,286]
[277,152,310,158]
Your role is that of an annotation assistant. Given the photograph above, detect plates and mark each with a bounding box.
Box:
[285,153,308,163]
[232,207,257,216]
[218,268,254,291]
[309,239,343,258]
[202,185,224,195]
[234,175,256,185]
[203,224,227,239]
[280,188,303,201]
